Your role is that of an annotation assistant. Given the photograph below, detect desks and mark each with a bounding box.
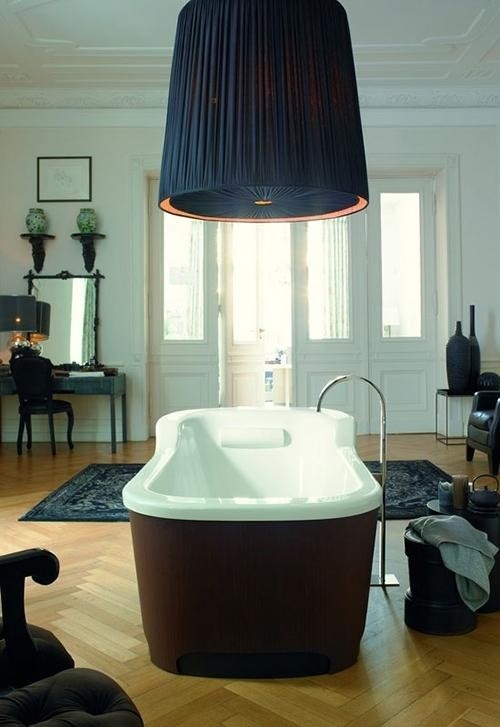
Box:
[435,389,472,446]
[0,369,127,454]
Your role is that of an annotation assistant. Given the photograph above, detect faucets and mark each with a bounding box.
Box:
[315,372,400,587]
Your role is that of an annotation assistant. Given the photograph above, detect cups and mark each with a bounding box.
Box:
[453,473,469,509]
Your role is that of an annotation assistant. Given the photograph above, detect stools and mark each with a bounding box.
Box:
[403,530,479,636]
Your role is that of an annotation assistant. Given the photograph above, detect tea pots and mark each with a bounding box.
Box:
[468,473,499,507]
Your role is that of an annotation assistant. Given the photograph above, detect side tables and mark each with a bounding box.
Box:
[426,499,499,615]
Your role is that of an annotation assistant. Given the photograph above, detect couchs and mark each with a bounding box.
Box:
[464,390,499,476]
[0,545,145,727]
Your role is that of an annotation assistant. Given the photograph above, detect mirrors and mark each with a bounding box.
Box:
[24,271,105,371]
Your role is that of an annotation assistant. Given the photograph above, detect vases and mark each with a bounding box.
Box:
[77,208,97,232]
[444,304,480,394]
[25,208,48,234]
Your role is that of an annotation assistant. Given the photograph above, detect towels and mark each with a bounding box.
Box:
[404,513,499,613]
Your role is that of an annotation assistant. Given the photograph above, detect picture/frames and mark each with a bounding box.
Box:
[37,156,91,203]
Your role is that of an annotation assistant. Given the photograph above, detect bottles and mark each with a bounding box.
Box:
[262,359,282,403]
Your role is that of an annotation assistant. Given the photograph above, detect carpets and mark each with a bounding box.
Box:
[19,461,459,525]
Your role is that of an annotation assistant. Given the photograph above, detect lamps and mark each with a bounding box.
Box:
[0,294,37,358]
[155,0,371,223]
[31,300,51,357]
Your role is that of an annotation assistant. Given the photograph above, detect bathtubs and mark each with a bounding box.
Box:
[121,405,384,680]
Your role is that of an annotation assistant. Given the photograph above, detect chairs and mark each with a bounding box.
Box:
[9,357,74,457]
